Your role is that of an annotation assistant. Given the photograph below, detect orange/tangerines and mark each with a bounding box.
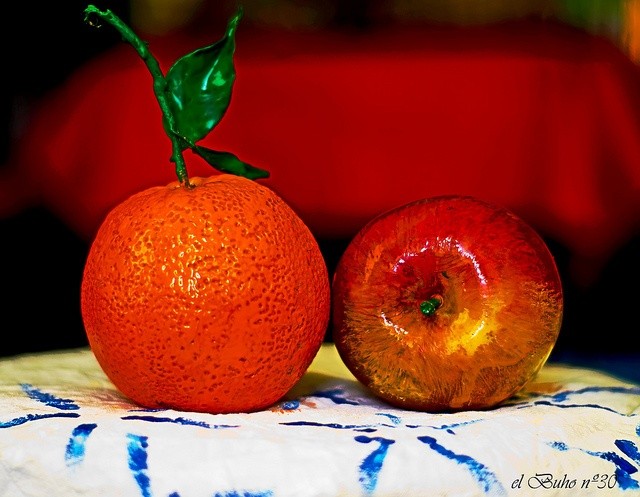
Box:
[77,173,332,417]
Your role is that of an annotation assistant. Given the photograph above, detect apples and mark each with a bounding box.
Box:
[328,193,564,415]
